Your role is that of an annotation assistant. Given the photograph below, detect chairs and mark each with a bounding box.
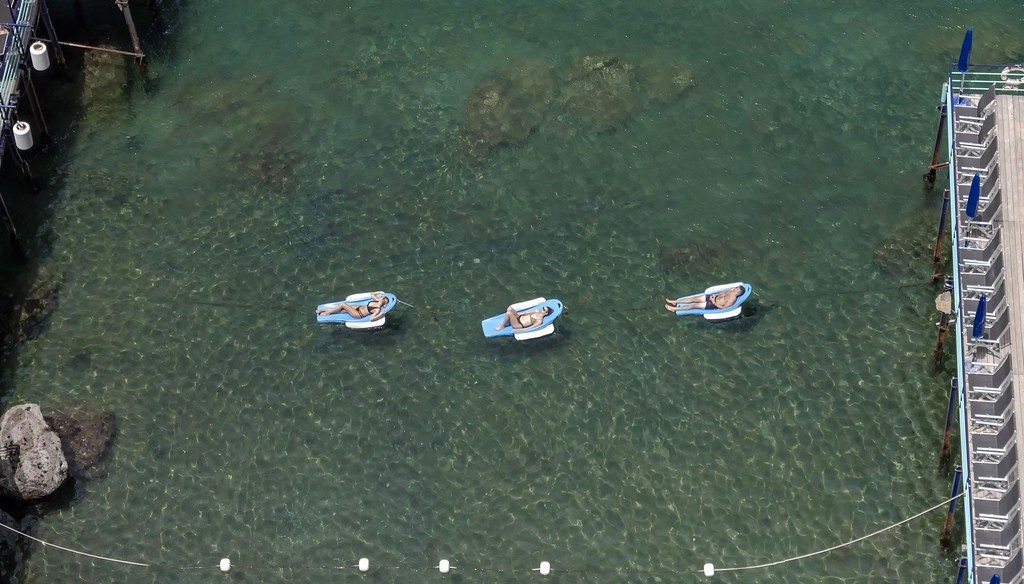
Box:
[955,85,1024,584]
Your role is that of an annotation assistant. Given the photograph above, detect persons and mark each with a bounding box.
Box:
[316,293,387,322]
[665,285,744,312]
[495,306,554,331]
